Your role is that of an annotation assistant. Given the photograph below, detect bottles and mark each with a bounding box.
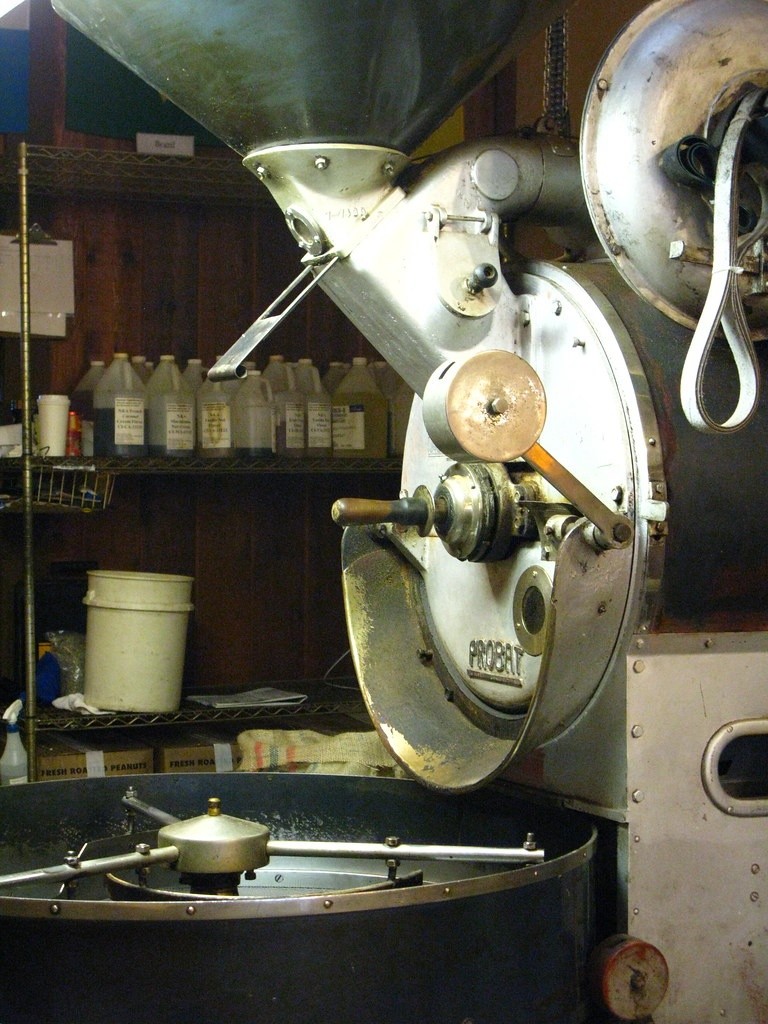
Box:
[4,399,23,424]
[67,352,242,460]
[232,355,414,461]
[0,724,28,787]
[67,411,94,457]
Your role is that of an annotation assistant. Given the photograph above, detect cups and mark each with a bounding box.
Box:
[0,423,22,458]
[38,641,53,660]
[37,395,70,457]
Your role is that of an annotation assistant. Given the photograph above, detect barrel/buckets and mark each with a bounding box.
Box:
[82,569,194,715]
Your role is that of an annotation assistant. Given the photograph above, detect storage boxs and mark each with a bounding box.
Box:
[149,727,245,773]
[24,730,156,783]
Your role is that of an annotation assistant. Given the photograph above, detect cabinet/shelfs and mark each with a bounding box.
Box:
[0,138,412,783]
